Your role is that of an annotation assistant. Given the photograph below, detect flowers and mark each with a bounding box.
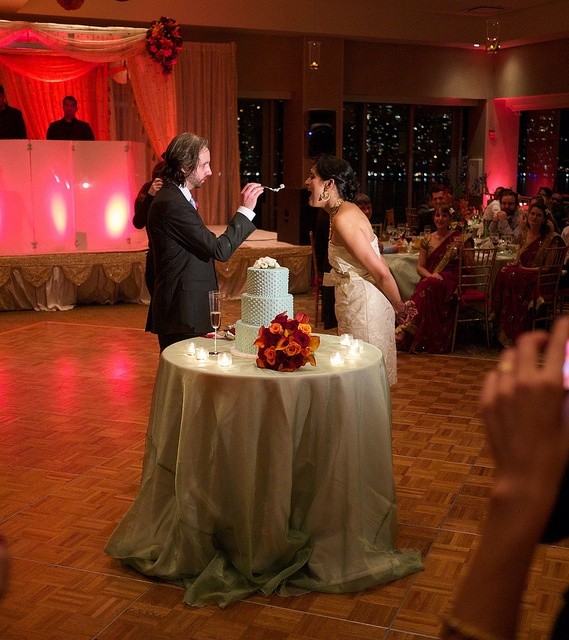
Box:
[460,200,479,221]
[146,16,184,74]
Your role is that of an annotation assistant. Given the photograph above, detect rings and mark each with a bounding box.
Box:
[493,360,517,374]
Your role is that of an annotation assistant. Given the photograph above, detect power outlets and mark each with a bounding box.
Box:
[100,332,426,609]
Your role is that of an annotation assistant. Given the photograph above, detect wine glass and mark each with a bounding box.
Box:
[397,224,405,245]
[424,225,432,239]
[405,227,413,253]
[386,226,395,247]
[501,235,513,254]
[208,290,222,355]
[490,233,500,255]
[508,239,518,258]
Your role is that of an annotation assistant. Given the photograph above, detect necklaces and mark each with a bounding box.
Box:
[328,198,346,240]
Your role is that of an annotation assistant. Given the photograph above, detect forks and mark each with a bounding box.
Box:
[257,184,286,194]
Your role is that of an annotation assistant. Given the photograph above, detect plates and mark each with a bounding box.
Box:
[230,346,261,360]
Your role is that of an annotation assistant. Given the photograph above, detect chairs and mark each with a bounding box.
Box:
[440,245,497,354]
[379,238,517,350]
[386,208,395,227]
[308,231,322,329]
[371,223,382,236]
[406,213,419,229]
[405,207,416,215]
[531,243,569,332]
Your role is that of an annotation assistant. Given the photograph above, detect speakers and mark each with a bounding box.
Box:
[303,108,336,159]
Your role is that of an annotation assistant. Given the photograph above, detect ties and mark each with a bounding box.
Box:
[187,197,193,207]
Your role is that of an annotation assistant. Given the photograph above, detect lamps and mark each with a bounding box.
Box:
[485,17,501,55]
[307,40,321,71]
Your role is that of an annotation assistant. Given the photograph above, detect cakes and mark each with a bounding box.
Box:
[236,255,295,354]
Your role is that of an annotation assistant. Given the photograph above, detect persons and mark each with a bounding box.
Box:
[304,153,418,387]
[561,226,569,277]
[0,85,27,139]
[351,191,383,255]
[147,130,264,360]
[395,203,478,354]
[547,194,564,214]
[488,203,559,349]
[416,193,434,214]
[488,191,528,244]
[442,187,452,204]
[476,187,505,238]
[526,194,562,235]
[414,187,469,234]
[46,96,94,140]
[435,313,569,640]
[133,162,168,298]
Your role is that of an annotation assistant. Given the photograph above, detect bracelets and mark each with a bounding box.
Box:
[436,611,506,640]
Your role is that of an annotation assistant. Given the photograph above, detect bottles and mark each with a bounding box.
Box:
[474,210,478,225]
[470,207,475,224]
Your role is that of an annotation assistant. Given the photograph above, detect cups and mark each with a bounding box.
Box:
[196,348,209,361]
[352,339,365,351]
[330,351,345,367]
[348,347,360,359]
[340,333,354,346]
[217,352,232,368]
[184,342,198,356]
